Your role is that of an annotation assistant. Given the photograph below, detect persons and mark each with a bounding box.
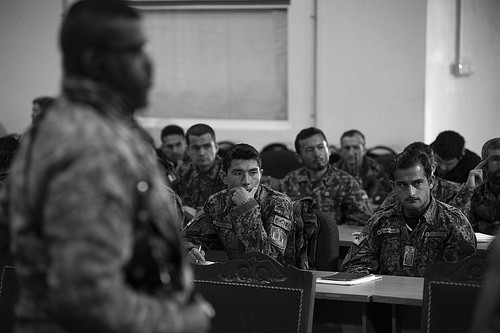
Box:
[170,124,229,220]
[0,137,21,185]
[178,143,308,271]
[422,130,483,183]
[454,137,500,235]
[331,130,386,195]
[31,97,54,124]
[279,128,374,228]
[377,141,459,211]
[339,151,477,276]
[159,125,191,174]
[8,0,212,333]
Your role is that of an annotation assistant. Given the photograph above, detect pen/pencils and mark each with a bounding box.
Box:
[195,245,204,264]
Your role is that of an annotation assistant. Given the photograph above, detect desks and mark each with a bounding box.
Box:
[309,224,495,332]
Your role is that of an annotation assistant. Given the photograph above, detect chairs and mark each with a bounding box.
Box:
[190,251,316,333]
[420,248,487,333]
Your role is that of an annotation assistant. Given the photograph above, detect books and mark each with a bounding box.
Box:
[315,269,381,286]
